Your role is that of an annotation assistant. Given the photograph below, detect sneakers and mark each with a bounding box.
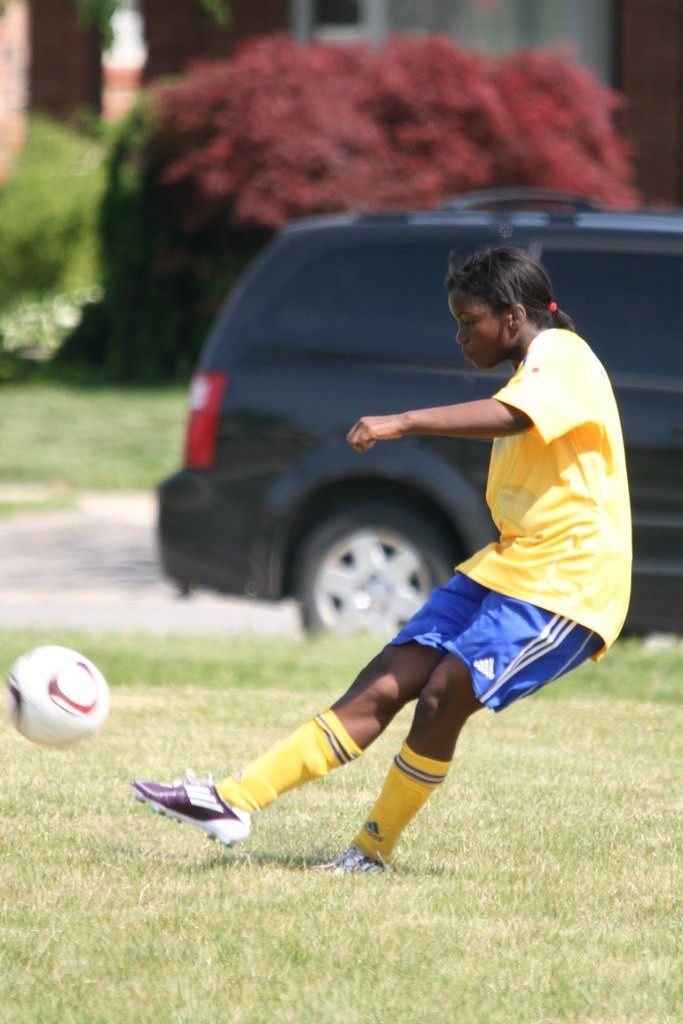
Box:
[134,771,255,851]
[306,847,388,880]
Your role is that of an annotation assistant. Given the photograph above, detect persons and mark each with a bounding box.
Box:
[128,245,636,880]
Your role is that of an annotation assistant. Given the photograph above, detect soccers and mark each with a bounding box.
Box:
[3,643,109,749]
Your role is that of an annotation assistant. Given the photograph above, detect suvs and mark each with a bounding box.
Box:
[155,182,681,641]
